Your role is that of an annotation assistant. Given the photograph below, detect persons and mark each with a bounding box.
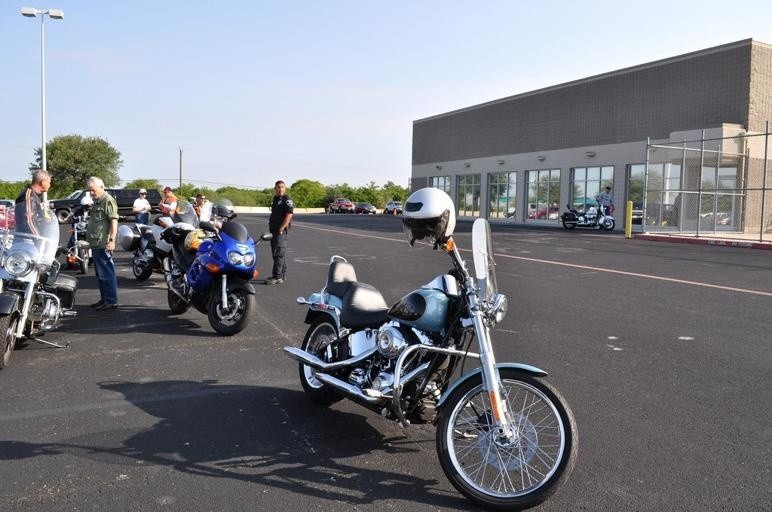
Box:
[192,193,214,221]
[264,181,293,284]
[673,190,681,226]
[159,187,178,219]
[598,187,615,215]
[132,188,151,232]
[12,169,51,244]
[85,176,119,311]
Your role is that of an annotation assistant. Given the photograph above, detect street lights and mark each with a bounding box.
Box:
[20,7,65,168]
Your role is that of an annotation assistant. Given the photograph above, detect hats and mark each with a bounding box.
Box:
[161,187,172,192]
[139,188,147,194]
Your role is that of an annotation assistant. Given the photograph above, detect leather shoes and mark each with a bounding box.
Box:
[95,302,118,311]
[90,299,104,308]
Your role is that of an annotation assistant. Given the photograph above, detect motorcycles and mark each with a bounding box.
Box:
[117,199,218,280]
[161,221,274,336]
[64,206,93,274]
[213,199,237,222]
[0,203,78,367]
[561,204,615,230]
[283,218,580,510]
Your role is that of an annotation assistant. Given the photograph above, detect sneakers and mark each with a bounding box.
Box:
[265,275,287,286]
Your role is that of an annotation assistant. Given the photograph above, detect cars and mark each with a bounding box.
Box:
[0,200,16,207]
[632,202,730,226]
[529,209,547,219]
[355,202,376,214]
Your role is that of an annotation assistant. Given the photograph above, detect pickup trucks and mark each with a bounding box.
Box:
[329,198,354,213]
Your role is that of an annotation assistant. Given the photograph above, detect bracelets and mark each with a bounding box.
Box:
[109,238,115,242]
[280,226,283,229]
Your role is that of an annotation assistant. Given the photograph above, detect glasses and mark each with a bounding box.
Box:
[200,198,207,199]
[140,193,147,195]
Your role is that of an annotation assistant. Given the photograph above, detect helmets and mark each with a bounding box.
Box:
[402,187,456,251]
[212,198,237,218]
[184,229,218,250]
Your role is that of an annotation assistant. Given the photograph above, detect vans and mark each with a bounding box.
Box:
[527,203,541,216]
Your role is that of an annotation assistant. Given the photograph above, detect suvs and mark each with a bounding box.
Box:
[503,206,515,218]
[49,189,164,224]
[385,202,402,214]
